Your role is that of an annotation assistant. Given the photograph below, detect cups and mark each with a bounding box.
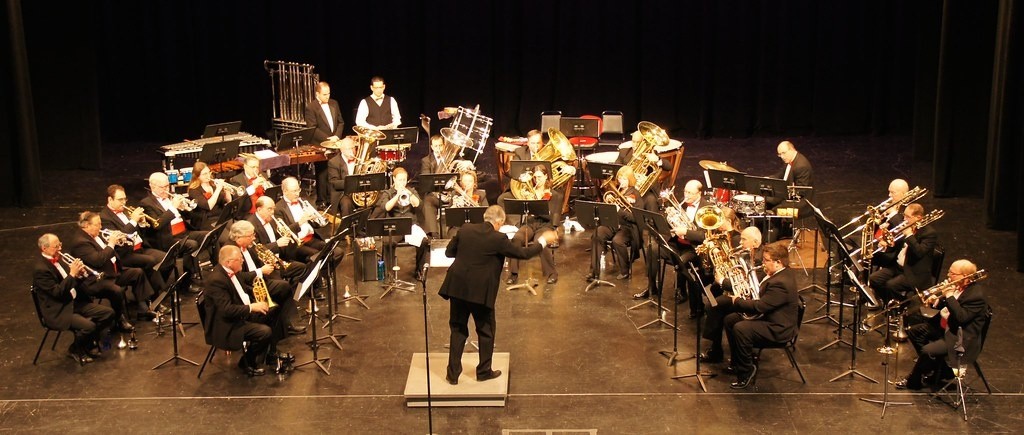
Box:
[165,169,178,184]
[180,167,193,182]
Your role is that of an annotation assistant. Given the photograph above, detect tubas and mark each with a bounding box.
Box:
[352,125,387,207]
[510,128,577,199]
[695,205,766,321]
[435,128,480,207]
[599,120,671,214]
[660,186,698,240]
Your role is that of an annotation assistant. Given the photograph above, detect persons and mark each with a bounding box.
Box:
[614,132,672,234]
[766,142,812,237]
[356,78,402,130]
[381,169,429,279]
[699,226,766,362]
[205,245,295,375]
[421,135,464,244]
[585,167,642,280]
[894,260,986,390]
[633,180,742,318]
[510,130,578,222]
[327,138,390,220]
[442,170,490,237]
[437,205,556,385]
[869,204,936,319]
[725,244,798,388]
[506,165,564,283]
[307,82,343,199]
[849,179,910,292]
[67,156,347,335]
[33,233,114,363]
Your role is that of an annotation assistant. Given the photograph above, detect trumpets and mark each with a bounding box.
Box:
[57,170,274,284]
[396,183,410,207]
[252,197,330,312]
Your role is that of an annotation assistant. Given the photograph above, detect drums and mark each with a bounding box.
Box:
[375,145,407,163]
[732,194,765,215]
[711,187,738,206]
[618,138,685,203]
[582,150,620,191]
[449,106,493,155]
[494,142,522,193]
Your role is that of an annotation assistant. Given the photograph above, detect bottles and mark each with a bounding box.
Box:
[103,330,112,350]
[343,285,350,298]
[149,300,152,310]
[378,259,385,280]
[662,311,667,322]
[829,252,834,266]
[564,216,571,234]
[116,327,139,351]
[600,251,607,268]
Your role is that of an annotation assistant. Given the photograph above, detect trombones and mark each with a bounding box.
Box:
[829,186,988,332]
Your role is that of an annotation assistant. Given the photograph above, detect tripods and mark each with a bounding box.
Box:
[560,115,718,393]
[928,326,979,421]
[293,206,417,376]
[783,185,914,419]
[151,235,201,369]
[504,198,549,296]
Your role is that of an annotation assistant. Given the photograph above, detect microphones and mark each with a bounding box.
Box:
[421,263,429,284]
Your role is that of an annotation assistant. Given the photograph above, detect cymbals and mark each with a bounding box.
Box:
[320,141,356,149]
[698,160,740,174]
[787,185,812,190]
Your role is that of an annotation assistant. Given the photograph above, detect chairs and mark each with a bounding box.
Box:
[540,111,562,145]
[31,189,564,379]
[568,114,603,154]
[883,243,992,395]
[599,111,625,147]
[600,231,807,393]
[799,189,819,218]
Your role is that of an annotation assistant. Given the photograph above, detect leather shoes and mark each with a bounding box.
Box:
[65,261,203,363]
[236,269,424,376]
[446,370,500,382]
[923,371,937,383]
[506,262,756,388]
[896,377,912,388]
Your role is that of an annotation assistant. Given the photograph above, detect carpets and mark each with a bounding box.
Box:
[765,229,829,268]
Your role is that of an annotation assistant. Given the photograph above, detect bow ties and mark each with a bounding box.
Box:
[765,269,773,277]
[955,285,962,291]
[226,272,235,278]
[291,200,300,205]
[241,246,246,252]
[375,97,382,101]
[687,198,701,208]
[320,102,327,104]
[263,221,268,225]
[114,208,125,214]
[48,256,59,264]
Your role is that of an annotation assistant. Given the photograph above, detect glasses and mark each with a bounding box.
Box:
[777,149,790,157]
[246,165,260,168]
[283,188,301,193]
[154,185,171,189]
[948,269,963,276]
[46,243,62,249]
[199,171,213,176]
[114,198,128,202]
